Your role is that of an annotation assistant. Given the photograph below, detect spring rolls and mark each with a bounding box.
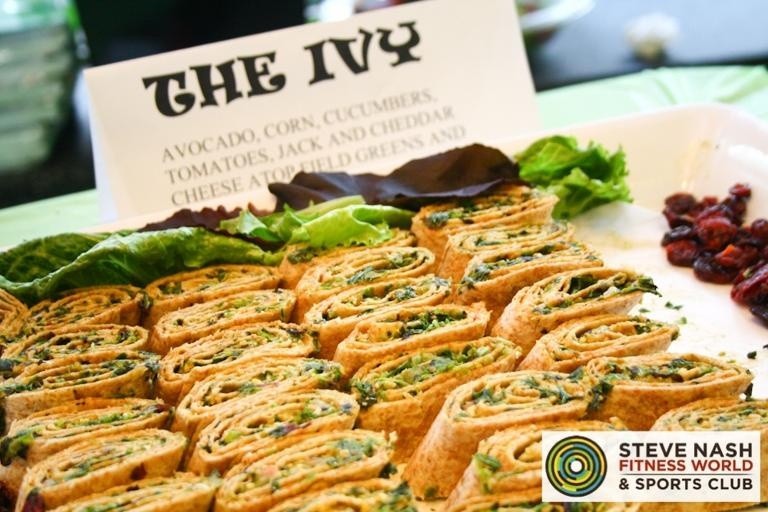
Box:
[0,184,768,512]
